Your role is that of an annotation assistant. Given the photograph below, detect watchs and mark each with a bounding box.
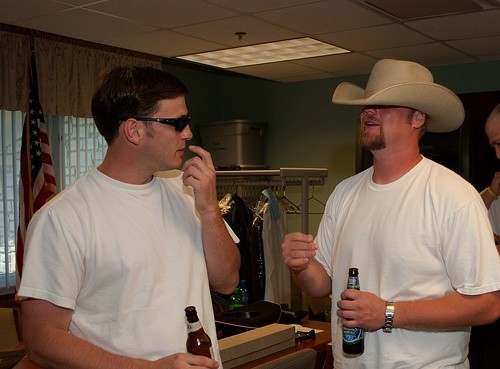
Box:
[382,301,394,333]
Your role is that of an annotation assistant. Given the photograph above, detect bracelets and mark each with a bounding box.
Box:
[488,187,498,199]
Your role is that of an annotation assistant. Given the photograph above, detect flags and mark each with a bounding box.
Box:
[16,52,56,295]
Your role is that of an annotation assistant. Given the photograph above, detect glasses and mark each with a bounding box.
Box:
[117,113,190,132]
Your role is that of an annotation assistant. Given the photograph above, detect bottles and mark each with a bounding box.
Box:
[239,280,248,304]
[185,306,215,361]
[230,288,243,311]
[342,268,364,355]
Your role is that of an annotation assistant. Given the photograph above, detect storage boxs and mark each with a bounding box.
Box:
[200,119,270,169]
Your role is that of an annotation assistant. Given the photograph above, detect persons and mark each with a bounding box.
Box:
[281,58,500,369]
[15,65,240,369]
[467,102,500,369]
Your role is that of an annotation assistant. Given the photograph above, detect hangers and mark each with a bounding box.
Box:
[216,179,326,215]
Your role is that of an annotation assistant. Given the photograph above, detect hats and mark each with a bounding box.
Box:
[332,58,464,131]
